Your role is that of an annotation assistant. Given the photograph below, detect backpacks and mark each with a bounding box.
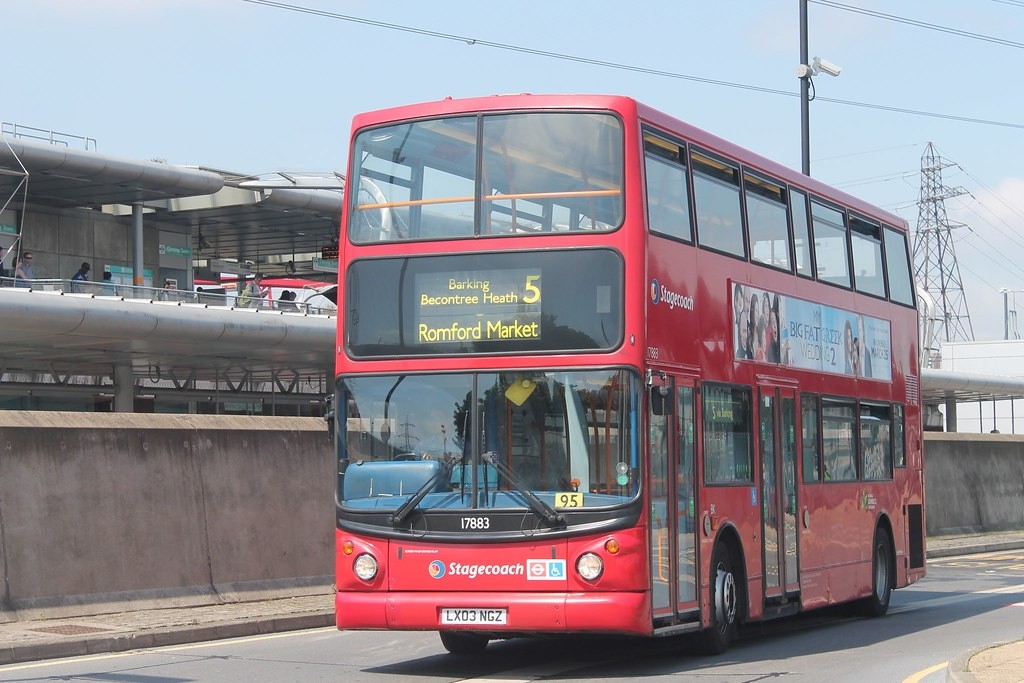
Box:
[239,281,253,308]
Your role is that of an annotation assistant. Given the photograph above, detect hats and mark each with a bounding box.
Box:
[82,262,91,271]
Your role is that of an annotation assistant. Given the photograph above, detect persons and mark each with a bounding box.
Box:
[18,252,34,288]
[844,319,854,375]
[852,336,863,376]
[0,247,7,287]
[857,313,873,378]
[246,273,270,310]
[70,261,90,293]
[9,256,31,288]
[95,271,116,296]
[404,403,464,463]
[287,291,301,313]
[159,281,171,301]
[277,289,290,310]
[196,287,207,304]
[731,282,791,364]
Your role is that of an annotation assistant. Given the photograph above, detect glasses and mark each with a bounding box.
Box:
[26,257,33,259]
[429,416,445,421]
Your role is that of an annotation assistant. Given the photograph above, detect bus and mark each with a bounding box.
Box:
[323,93,928,655]
